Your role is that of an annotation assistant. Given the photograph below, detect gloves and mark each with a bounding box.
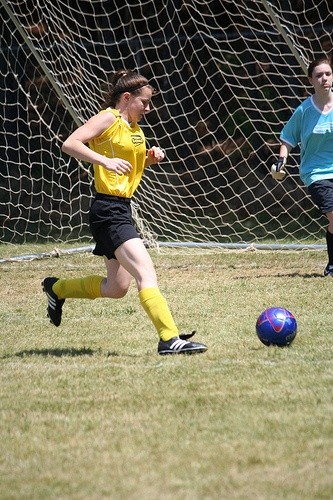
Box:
[271,156,287,180]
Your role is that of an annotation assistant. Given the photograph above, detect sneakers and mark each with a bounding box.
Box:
[156,330,208,355]
[41,276,63,328]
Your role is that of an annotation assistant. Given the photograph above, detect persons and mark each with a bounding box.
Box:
[271,61,333,276]
[42,70,208,355]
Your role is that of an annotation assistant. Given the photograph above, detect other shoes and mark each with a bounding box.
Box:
[324,262,333,277]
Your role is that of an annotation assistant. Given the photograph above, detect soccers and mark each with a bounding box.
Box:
[254,307,297,348]
